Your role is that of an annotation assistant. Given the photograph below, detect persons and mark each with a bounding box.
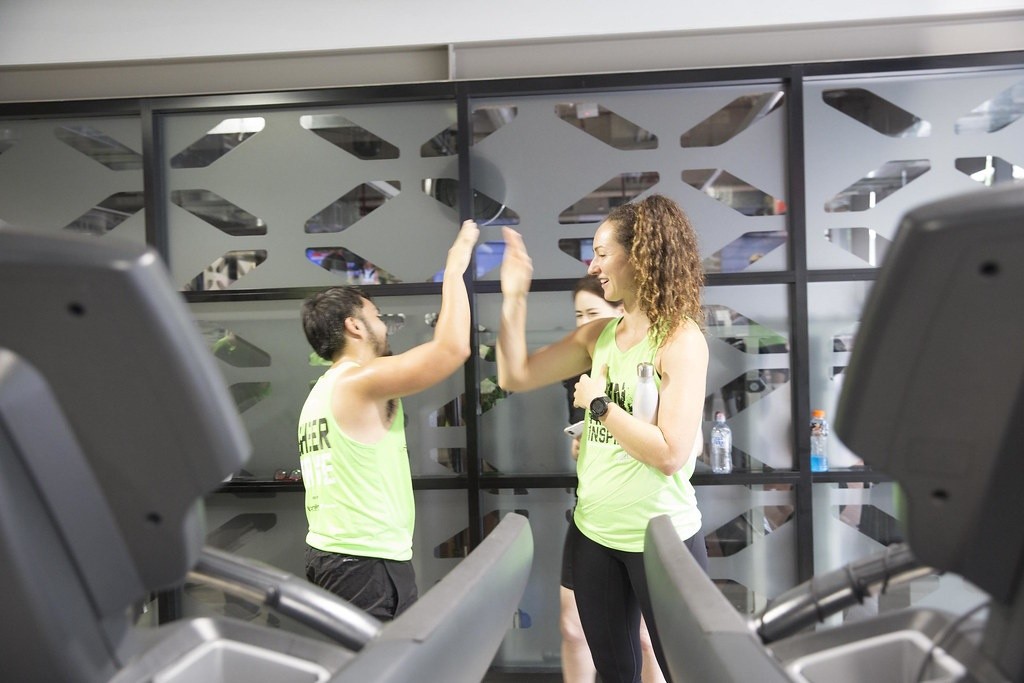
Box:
[559,274,666,683]
[495,193,709,683]
[298,219,480,623]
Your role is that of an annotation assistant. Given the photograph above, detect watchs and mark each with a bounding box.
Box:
[589,396,613,421]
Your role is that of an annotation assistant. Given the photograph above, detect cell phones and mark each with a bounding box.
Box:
[564,420,583,439]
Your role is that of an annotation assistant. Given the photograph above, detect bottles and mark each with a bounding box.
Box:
[711,413,733,474]
[810,411,828,472]
